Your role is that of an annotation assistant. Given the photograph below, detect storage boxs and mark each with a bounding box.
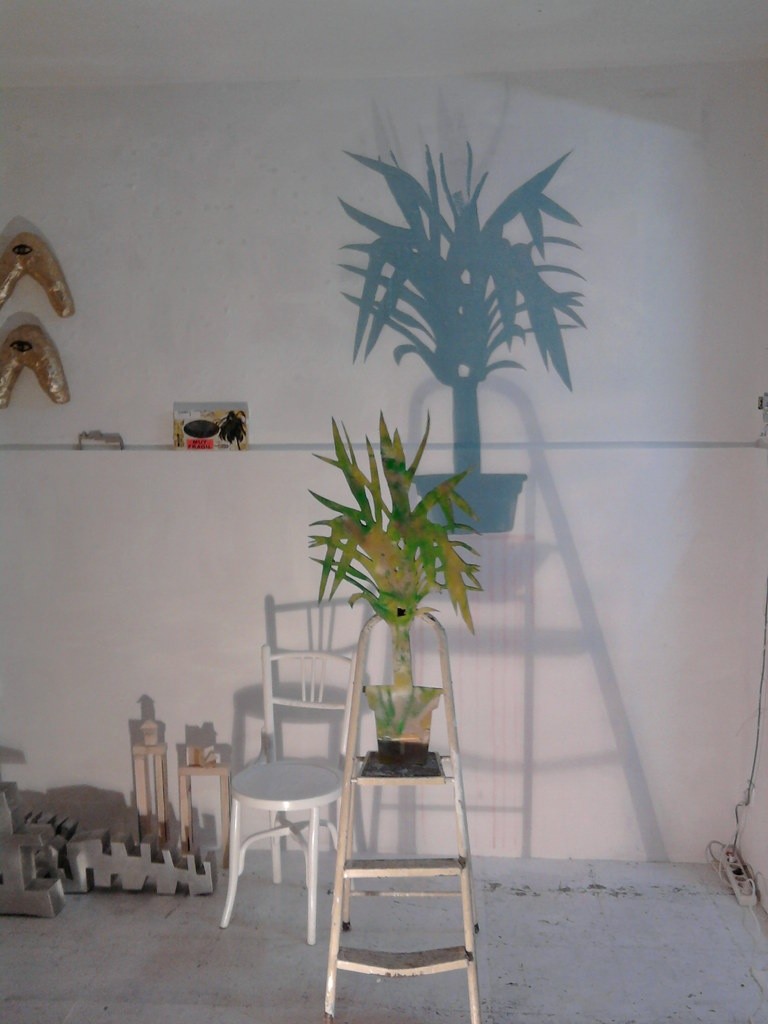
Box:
[171,401,250,451]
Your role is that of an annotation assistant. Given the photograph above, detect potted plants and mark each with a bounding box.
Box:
[304,404,493,772]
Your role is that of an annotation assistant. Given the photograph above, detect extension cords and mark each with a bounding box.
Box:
[720,845,757,907]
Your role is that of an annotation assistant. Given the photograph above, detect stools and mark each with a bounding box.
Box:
[176,763,233,868]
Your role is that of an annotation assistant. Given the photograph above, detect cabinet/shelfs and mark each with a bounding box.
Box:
[320,600,483,1024]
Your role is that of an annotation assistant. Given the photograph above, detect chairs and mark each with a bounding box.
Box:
[214,636,357,948]
